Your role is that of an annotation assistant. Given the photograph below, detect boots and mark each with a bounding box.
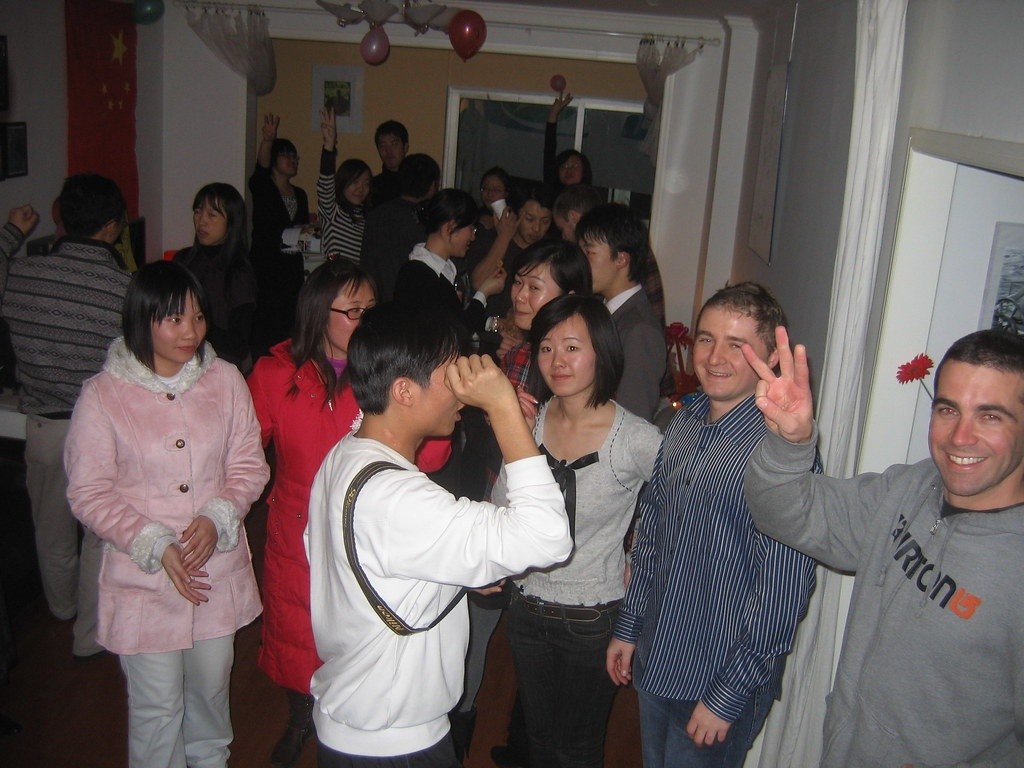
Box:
[448,705,477,764]
[270,689,315,768]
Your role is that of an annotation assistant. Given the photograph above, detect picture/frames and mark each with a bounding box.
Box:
[978,221,1024,338]
[311,63,365,135]
[0,121,29,178]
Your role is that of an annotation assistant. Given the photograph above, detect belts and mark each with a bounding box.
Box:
[38,410,73,420]
[510,582,621,622]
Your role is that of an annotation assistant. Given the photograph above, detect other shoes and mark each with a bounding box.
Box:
[0,719,23,737]
[73,649,108,663]
[490,744,529,768]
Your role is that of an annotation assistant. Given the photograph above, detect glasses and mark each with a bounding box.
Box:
[466,225,477,234]
[479,187,506,196]
[105,219,129,231]
[279,152,300,162]
[329,303,375,320]
[560,162,584,169]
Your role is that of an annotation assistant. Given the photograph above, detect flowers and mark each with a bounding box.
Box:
[895,354,934,402]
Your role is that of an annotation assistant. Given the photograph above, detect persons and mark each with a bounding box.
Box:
[247,255,378,768]
[743,326,1024,768]
[448,238,592,768]
[302,306,574,768]
[489,293,665,768]
[606,281,824,768]
[64,259,271,768]
[249,89,676,499]
[0,173,132,660]
[171,181,257,366]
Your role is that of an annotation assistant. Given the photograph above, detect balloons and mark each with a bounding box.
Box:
[360,24,389,65]
[132,0,165,24]
[551,75,566,91]
[449,9,487,62]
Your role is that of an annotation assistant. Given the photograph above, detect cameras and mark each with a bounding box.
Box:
[467,578,511,610]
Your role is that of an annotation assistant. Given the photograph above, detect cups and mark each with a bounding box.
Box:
[490,198,510,220]
